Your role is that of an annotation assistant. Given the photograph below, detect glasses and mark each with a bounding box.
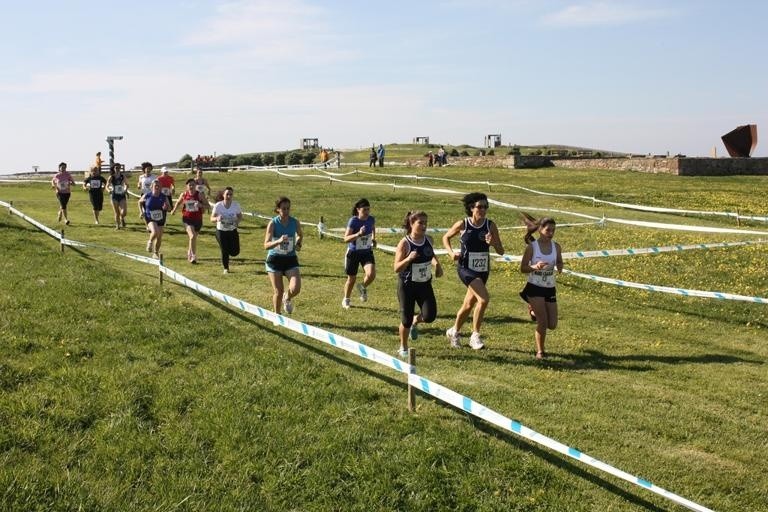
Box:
[475,205,488,209]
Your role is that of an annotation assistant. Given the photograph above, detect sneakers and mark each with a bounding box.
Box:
[147,241,158,259]
[58,212,69,224]
[224,269,230,275]
[282,293,292,314]
[188,252,196,264]
[528,305,536,321]
[399,349,408,357]
[410,324,417,340]
[445,328,461,349]
[357,282,367,301]
[96,219,99,224]
[116,219,124,230]
[342,299,350,309]
[536,352,547,359]
[469,338,485,349]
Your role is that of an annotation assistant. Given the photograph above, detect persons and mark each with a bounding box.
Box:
[138,162,158,232]
[428,151,434,166]
[341,198,377,311]
[82,165,107,225]
[171,178,210,264]
[369,148,378,167]
[320,147,329,169]
[51,161,76,226]
[263,196,304,316]
[392,208,443,358]
[95,151,103,175]
[378,144,385,167]
[437,145,447,167]
[210,186,243,275]
[519,211,564,360]
[441,192,505,353]
[434,154,440,164]
[191,168,212,214]
[105,163,130,231]
[157,166,175,214]
[137,178,171,259]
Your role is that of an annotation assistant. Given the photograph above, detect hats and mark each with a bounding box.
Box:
[161,167,168,172]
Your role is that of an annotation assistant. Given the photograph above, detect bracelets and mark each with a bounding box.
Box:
[355,233,361,238]
